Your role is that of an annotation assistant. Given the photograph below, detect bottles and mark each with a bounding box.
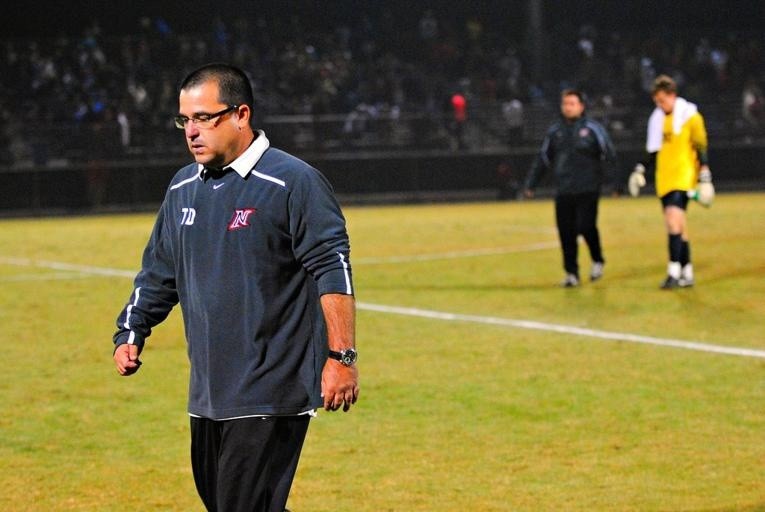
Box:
[687,191,713,208]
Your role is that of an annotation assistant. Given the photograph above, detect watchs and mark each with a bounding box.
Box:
[328,348,358,366]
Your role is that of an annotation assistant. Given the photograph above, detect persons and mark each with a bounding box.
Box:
[522,88,618,286]
[628,74,715,288]
[111,63,360,512]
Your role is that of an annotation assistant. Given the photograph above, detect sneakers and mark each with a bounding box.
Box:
[563,261,692,290]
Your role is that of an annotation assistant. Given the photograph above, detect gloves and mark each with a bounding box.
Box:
[694,170,717,208]
[628,163,647,198]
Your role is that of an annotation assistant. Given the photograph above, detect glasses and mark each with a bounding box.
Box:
[173,105,241,130]
